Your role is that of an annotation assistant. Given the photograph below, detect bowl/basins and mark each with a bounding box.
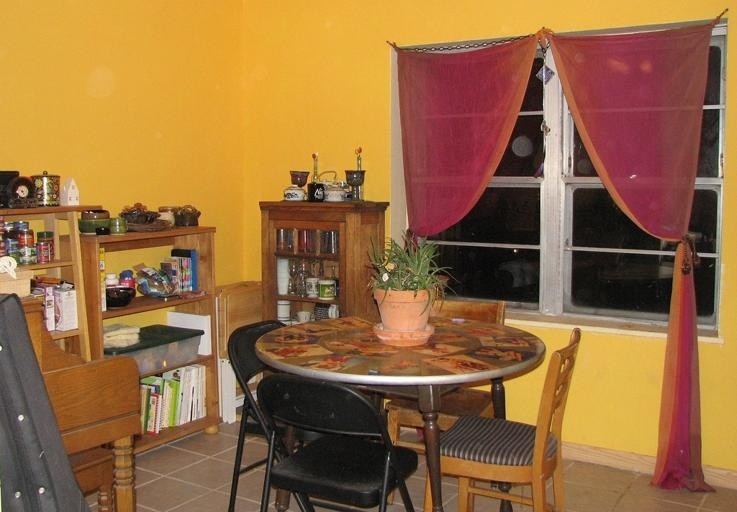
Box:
[106,287,136,307]
[80,210,110,220]
[121,211,161,225]
[127,219,173,233]
[173,216,199,227]
[324,184,346,202]
[77,219,113,233]
[283,184,305,201]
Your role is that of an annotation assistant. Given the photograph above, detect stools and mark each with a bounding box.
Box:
[216,280,263,424]
[68,445,115,511]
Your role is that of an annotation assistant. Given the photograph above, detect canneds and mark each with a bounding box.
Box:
[4,221,55,264]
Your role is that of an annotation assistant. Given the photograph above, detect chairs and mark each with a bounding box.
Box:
[256,374,416,512]
[382,294,507,511]
[420,325,583,511]
[227,319,324,512]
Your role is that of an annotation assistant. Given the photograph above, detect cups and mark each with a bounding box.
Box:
[275,227,339,254]
[111,216,129,234]
[305,277,337,300]
[307,182,324,202]
[275,299,339,326]
[288,169,309,187]
[287,258,311,298]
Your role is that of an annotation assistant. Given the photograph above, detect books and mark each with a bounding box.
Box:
[140,363,207,436]
[160,248,198,295]
[102,323,141,349]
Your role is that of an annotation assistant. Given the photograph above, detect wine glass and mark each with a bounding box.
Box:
[343,169,365,202]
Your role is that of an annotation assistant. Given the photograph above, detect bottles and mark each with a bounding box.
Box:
[31,170,61,207]
[105,271,136,287]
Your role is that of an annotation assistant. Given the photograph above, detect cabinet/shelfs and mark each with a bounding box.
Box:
[59,225,223,453]
[1,204,92,364]
[259,200,389,324]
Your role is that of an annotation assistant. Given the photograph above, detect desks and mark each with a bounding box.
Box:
[254,309,548,512]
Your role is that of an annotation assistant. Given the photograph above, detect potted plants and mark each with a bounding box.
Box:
[363,231,462,347]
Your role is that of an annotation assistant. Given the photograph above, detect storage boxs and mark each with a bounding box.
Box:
[103,336,166,376]
[140,322,205,369]
